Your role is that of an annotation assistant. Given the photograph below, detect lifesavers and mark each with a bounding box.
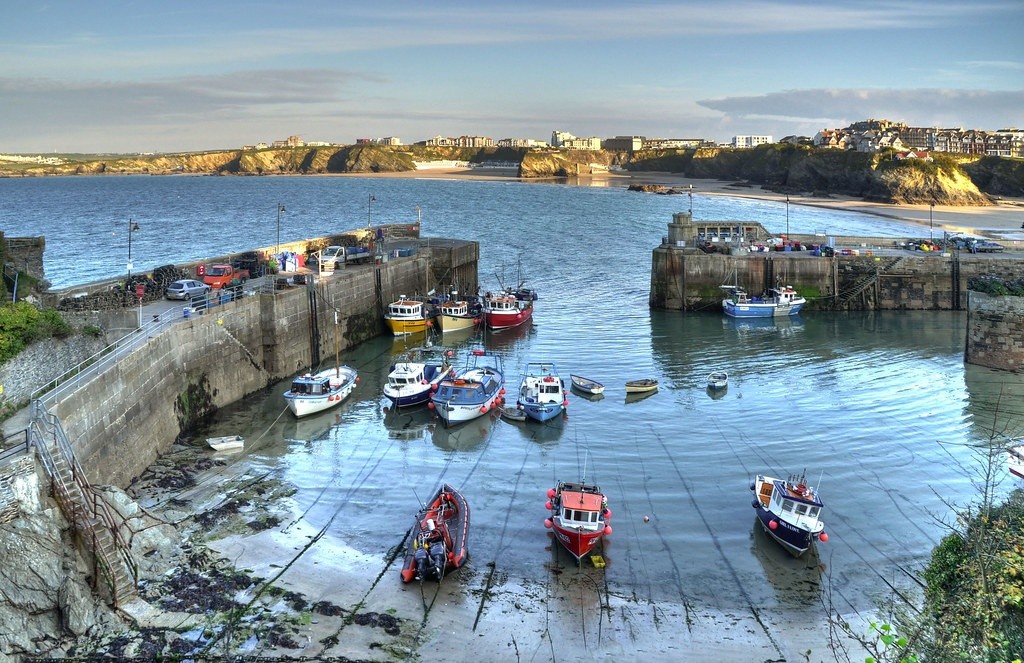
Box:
[313,376,322,380]
[543,377,554,382]
[299,376,305,380]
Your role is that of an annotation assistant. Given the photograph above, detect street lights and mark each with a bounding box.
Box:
[128,218,141,281]
[786,194,790,240]
[277,201,287,254]
[368,193,377,229]
[930,200,936,245]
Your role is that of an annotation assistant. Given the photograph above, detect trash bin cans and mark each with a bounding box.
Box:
[183,307,191,318]
[908,241,915,251]
[722,246,729,255]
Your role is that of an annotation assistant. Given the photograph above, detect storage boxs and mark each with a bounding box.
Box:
[751,235,872,256]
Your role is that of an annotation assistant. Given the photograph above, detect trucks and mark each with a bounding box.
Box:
[317,245,370,269]
[202,264,251,289]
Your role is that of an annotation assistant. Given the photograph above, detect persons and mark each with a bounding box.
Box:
[970,237,978,254]
[414,533,429,550]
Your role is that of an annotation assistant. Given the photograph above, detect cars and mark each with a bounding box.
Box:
[165,280,211,302]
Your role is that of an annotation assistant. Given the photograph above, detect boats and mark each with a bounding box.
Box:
[544,445,612,563]
[748,465,830,560]
[429,323,508,430]
[719,260,807,320]
[481,314,539,358]
[569,374,606,396]
[497,405,528,423]
[205,434,245,453]
[480,249,539,331]
[388,326,439,366]
[283,311,361,420]
[399,481,472,585]
[515,362,569,424]
[282,394,354,443]
[382,300,455,409]
[384,238,486,337]
[437,324,479,359]
[624,378,659,394]
[706,369,728,390]
[720,310,805,339]
